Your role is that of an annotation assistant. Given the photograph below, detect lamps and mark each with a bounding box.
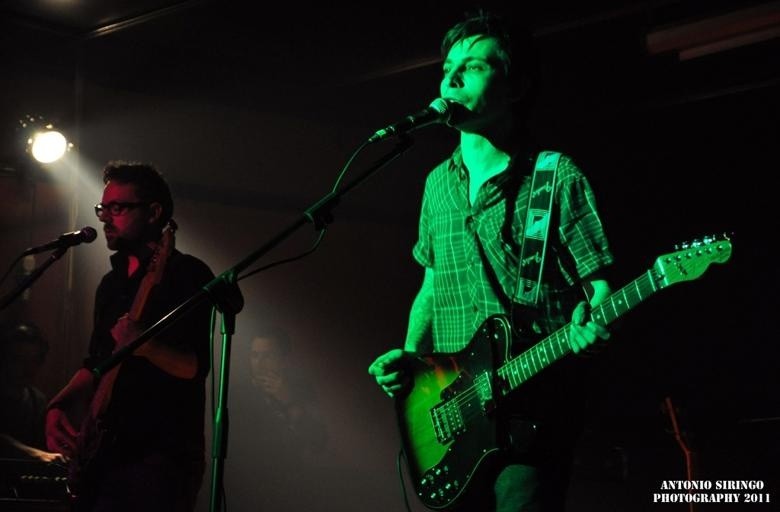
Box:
[14,113,71,165]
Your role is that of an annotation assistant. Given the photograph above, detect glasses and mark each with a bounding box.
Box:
[93,200,148,219]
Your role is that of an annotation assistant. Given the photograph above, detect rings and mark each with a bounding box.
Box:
[58,442,70,450]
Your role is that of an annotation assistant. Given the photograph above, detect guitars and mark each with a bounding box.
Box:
[394,231,733,511]
[64,227,175,511]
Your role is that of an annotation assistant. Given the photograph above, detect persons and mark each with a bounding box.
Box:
[362,20,635,512]
[41,155,215,510]
[1,320,73,469]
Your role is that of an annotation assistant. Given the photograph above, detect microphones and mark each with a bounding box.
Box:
[367,97,452,144]
[22,226,97,255]
